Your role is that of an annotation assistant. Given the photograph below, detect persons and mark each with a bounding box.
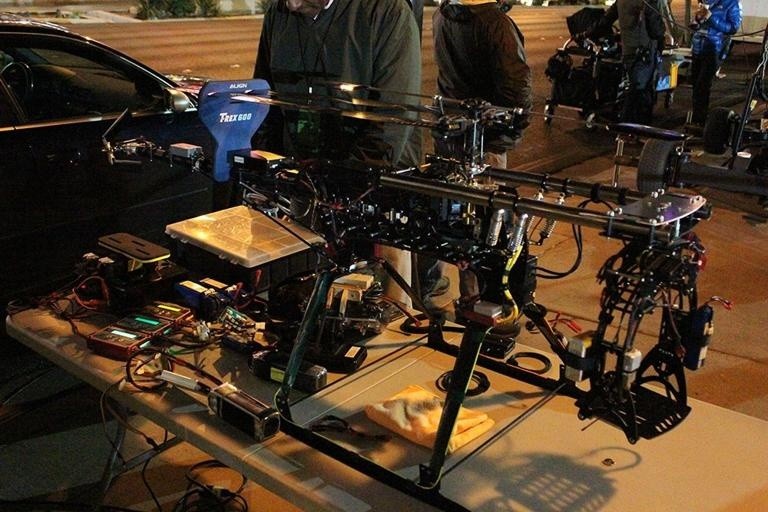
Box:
[253,0,422,193]
[686,1,743,123]
[434,0,532,196]
[580,1,667,133]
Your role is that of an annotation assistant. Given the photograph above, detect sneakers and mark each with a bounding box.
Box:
[380,297,409,319]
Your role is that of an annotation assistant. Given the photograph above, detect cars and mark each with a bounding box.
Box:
[0,10,233,306]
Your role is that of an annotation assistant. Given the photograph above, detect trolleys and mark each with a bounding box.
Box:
[544,38,600,128]
